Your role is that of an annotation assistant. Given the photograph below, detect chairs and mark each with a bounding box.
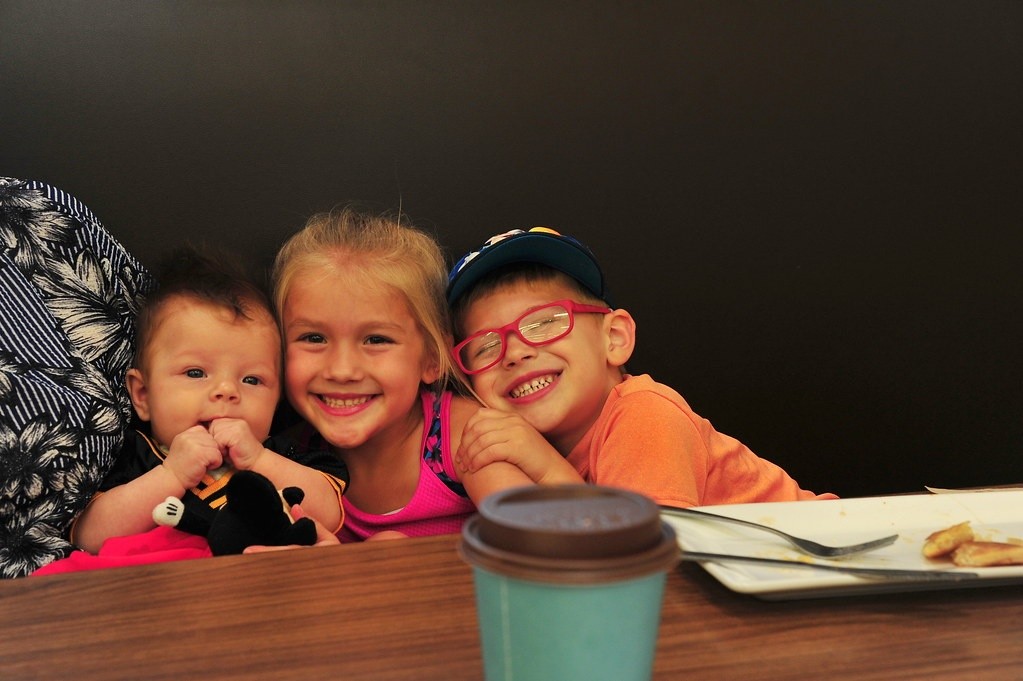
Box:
[0,176,168,583]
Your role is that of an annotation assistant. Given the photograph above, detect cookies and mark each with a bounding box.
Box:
[922,519,1023,568]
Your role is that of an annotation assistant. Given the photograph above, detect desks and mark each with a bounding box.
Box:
[0,488,1023,681]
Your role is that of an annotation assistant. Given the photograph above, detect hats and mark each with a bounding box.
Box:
[445,227,606,306]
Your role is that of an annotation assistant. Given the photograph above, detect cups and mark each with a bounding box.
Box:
[456,483,681,680]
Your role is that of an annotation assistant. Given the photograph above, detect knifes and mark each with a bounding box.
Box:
[680,548,979,581]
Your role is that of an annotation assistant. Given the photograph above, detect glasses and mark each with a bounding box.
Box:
[454,299,613,374]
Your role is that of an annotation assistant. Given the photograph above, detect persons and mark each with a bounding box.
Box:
[446,224,841,508]
[273,213,540,547]
[70,256,411,554]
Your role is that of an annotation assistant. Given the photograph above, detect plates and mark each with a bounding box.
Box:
[657,490,1023,593]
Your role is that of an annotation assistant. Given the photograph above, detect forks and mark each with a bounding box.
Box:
[658,504,899,557]
